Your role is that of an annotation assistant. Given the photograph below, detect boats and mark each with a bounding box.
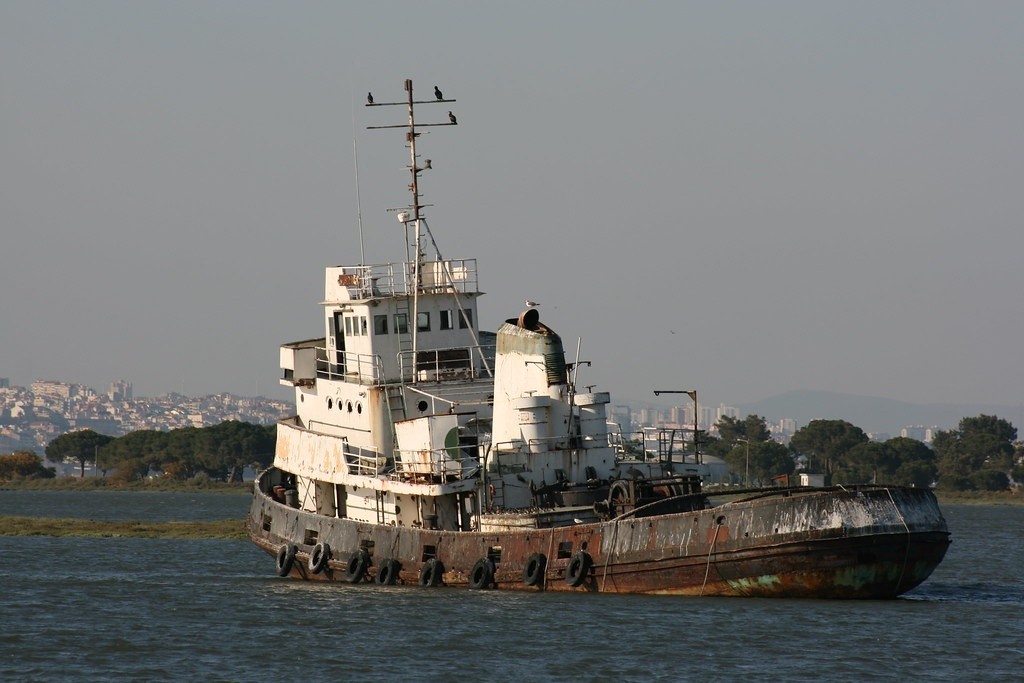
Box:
[248,79,954,604]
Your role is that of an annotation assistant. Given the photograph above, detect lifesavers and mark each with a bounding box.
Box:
[467,558,494,590]
[565,552,590,587]
[375,558,395,586]
[308,542,329,575]
[343,551,368,584]
[521,553,545,586]
[275,543,295,577]
[418,559,442,588]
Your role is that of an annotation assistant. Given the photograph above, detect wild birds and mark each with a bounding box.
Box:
[525,300,540,309]
[448,110,457,124]
[367,92,374,104]
[433,86,444,101]
